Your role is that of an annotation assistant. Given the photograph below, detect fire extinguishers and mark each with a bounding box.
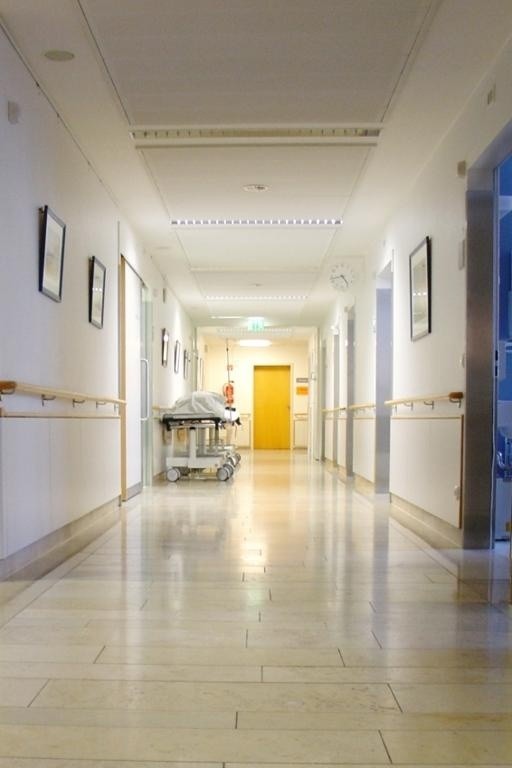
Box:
[223,380,235,402]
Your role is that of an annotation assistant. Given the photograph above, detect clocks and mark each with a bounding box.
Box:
[330,263,355,289]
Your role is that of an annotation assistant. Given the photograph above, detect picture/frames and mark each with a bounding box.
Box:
[89,256,106,329]
[184,351,187,379]
[162,328,168,368]
[174,340,180,374]
[409,236,431,341]
[39,204,66,303]
